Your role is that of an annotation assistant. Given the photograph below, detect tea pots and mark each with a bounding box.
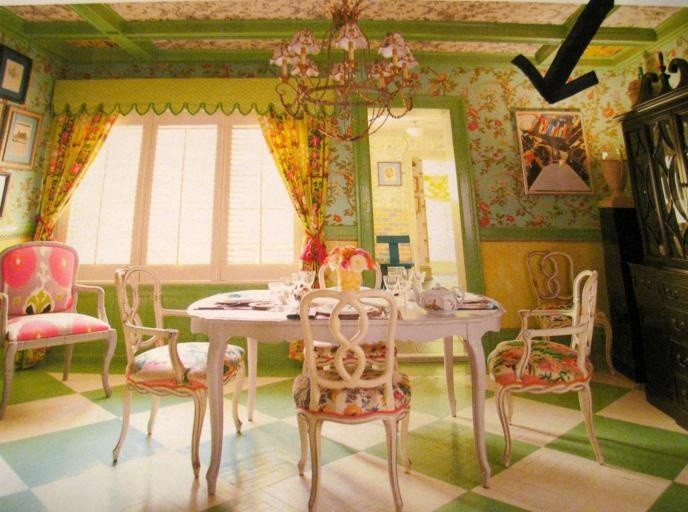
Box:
[410,281,466,315]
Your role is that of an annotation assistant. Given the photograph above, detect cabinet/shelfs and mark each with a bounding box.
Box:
[605,86,688,431]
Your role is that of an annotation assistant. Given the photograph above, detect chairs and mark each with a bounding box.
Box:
[1,240,121,408]
[300,245,402,365]
[483,266,609,470]
[108,265,247,483]
[290,288,418,512]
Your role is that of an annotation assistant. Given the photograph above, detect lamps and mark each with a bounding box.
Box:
[266,1,420,143]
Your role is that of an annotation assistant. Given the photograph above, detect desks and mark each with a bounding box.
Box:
[186,279,506,498]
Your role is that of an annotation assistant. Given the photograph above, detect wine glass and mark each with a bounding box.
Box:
[267,271,316,311]
[383,266,425,306]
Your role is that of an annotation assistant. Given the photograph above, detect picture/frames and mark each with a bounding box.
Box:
[514,109,595,196]
[377,160,402,187]
[0,44,44,171]
